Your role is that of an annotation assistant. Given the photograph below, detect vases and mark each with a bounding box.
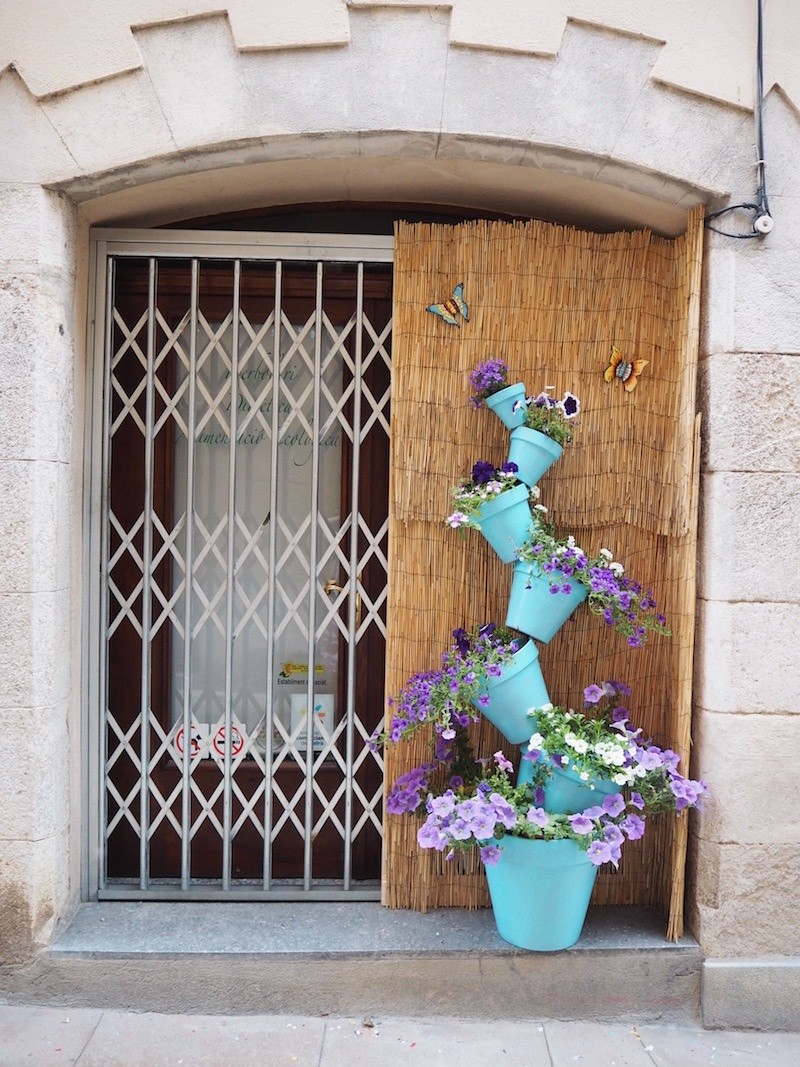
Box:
[505,560,588,645]
[472,483,534,565]
[506,426,563,487]
[483,834,599,952]
[485,382,527,431]
[515,743,623,816]
[470,638,552,744]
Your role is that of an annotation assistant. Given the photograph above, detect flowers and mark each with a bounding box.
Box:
[368,359,705,955]
